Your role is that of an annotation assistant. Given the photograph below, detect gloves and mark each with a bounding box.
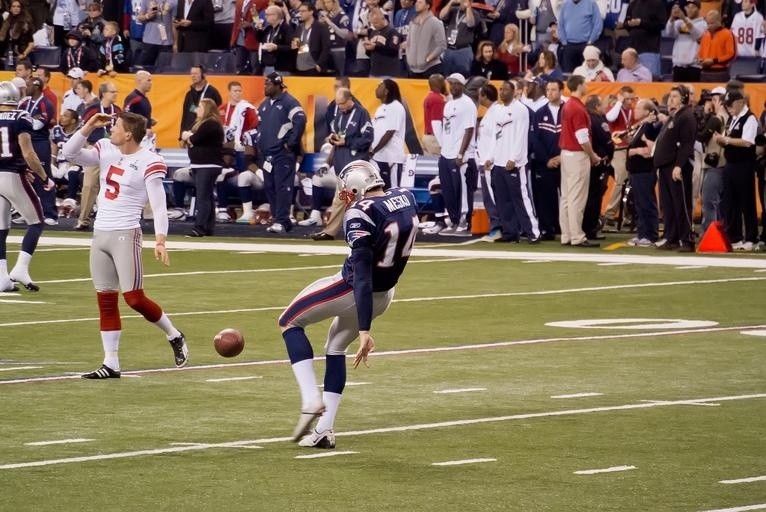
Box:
[41,176,57,193]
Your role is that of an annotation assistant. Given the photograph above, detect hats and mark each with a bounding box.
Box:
[66,66,85,81]
[446,72,466,86]
[266,71,288,89]
[707,86,727,96]
[582,43,602,62]
[720,89,744,106]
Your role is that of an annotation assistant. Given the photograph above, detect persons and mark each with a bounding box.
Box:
[277,158,420,449]
[0,80,55,293]
[61,110,190,381]
[0,1,766,253]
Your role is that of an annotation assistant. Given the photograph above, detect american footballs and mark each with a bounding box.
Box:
[214,329,245,357]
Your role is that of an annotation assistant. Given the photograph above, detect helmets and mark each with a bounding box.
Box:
[0,80,21,106]
[336,159,386,205]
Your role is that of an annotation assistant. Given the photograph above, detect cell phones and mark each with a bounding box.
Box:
[152,6,157,10]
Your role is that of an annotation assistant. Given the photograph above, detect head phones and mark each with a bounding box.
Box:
[678,86,689,104]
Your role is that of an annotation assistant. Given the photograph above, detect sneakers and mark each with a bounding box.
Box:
[289,398,327,442]
[419,218,606,248]
[166,329,189,368]
[0,278,20,293]
[732,240,764,252]
[72,220,91,232]
[8,268,40,292]
[80,364,122,380]
[626,236,695,252]
[168,207,333,241]
[297,427,336,449]
[43,216,60,226]
[12,217,26,225]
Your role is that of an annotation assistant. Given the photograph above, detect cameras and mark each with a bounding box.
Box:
[703,154,719,167]
[214,5,223,12]
